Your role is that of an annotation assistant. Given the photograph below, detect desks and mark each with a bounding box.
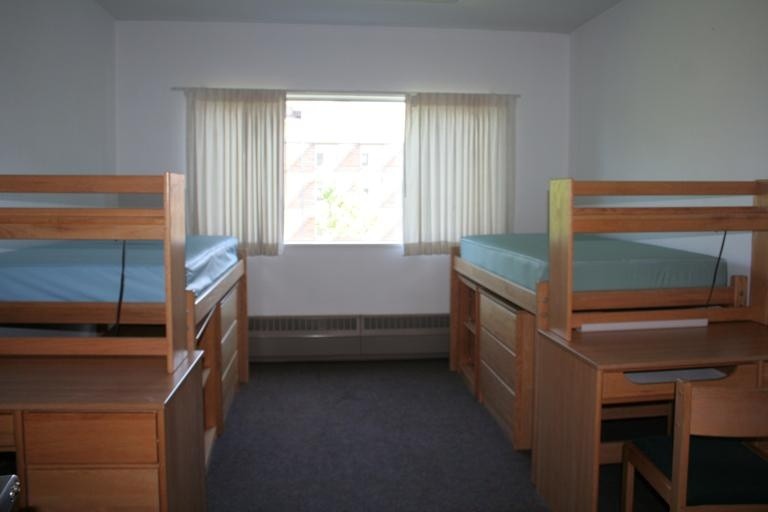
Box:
[534,320,768,511]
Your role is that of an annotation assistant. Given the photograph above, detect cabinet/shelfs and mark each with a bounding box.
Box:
[0,348,205,512]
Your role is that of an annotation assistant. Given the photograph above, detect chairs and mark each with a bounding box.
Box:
[621,359,768,511]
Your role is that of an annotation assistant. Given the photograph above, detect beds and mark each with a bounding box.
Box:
[0,173,249,435]
[449,176,768,452]
